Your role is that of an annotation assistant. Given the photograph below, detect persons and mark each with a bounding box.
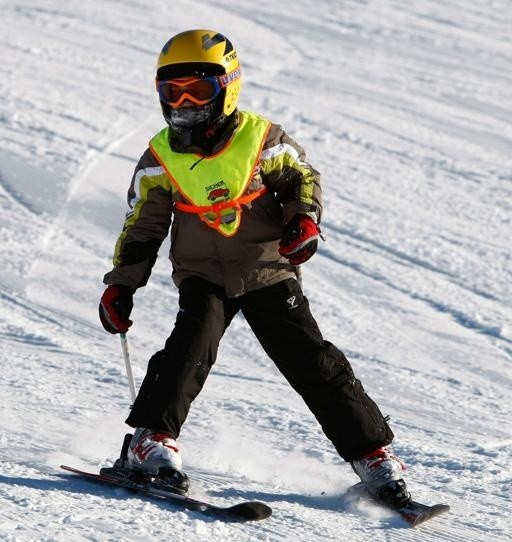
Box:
[98,29,411,508]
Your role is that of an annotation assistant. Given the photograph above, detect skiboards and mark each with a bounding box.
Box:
[60,433,451,526]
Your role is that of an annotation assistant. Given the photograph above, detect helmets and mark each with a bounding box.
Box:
[154,29,242,137]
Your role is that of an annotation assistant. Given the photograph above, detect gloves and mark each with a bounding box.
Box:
[278,216,319,266]
[98,286,133,334]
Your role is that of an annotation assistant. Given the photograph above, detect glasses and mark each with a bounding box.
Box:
[156,75,220,110]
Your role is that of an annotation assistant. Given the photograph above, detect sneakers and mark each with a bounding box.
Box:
[134,430,182,468]
[351,447,405,483]
